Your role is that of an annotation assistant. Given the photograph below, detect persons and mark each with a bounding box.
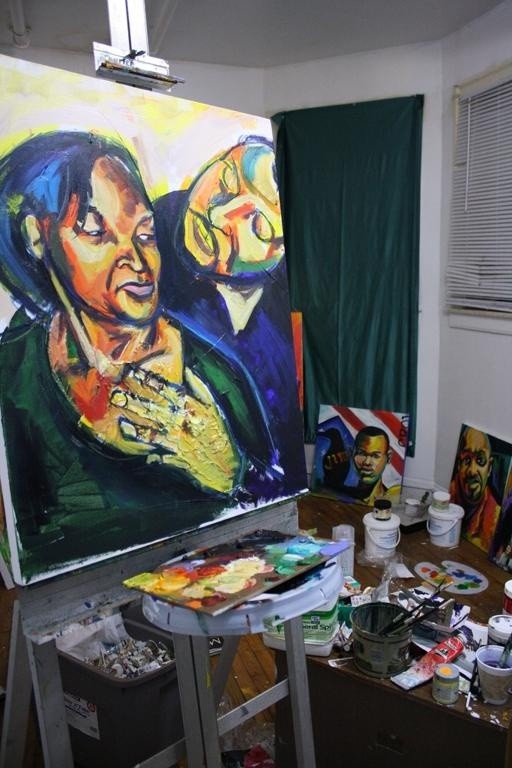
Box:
[449,426,501,555]
[151,135,308,488]
[352,426,403,508]
[1,131,287,574]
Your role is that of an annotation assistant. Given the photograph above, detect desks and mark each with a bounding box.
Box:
[271,598,511,767]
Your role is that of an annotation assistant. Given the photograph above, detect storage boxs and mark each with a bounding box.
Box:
[23,607,229,767]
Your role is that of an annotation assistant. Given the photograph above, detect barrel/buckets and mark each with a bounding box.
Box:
[487,613,512,646]
[362,512,401,558]
[426,503,465,548]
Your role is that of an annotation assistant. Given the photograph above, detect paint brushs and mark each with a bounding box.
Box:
[378,577,455,636]
[463,638,484,711]
